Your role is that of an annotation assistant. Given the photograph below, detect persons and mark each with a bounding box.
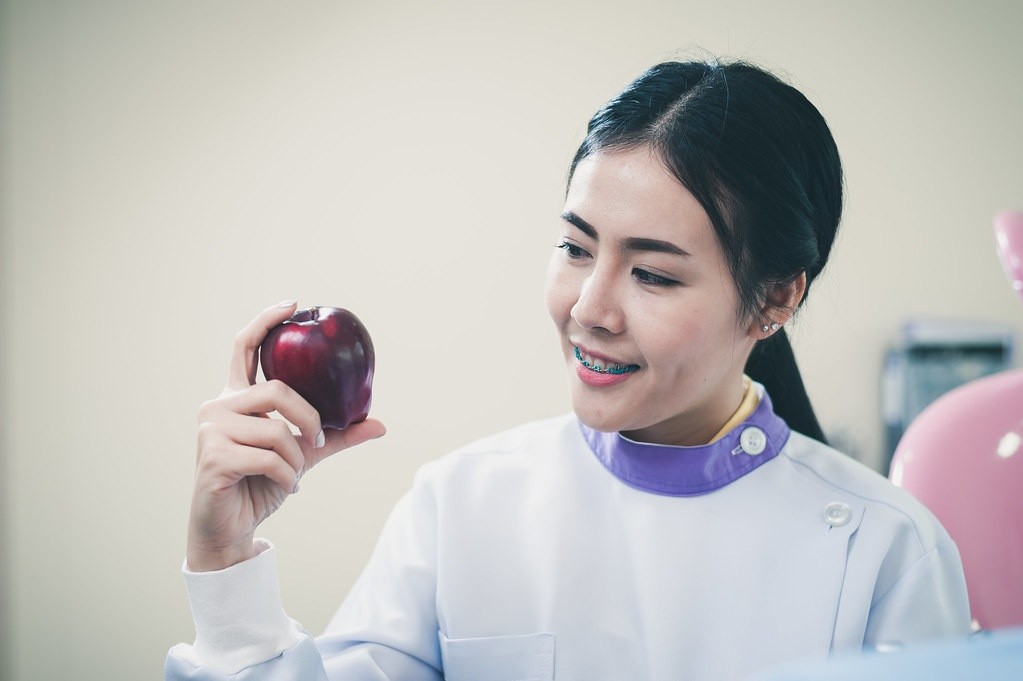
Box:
[163,63,972,681]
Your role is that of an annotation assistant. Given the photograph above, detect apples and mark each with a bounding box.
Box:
[259,306,376,430]
